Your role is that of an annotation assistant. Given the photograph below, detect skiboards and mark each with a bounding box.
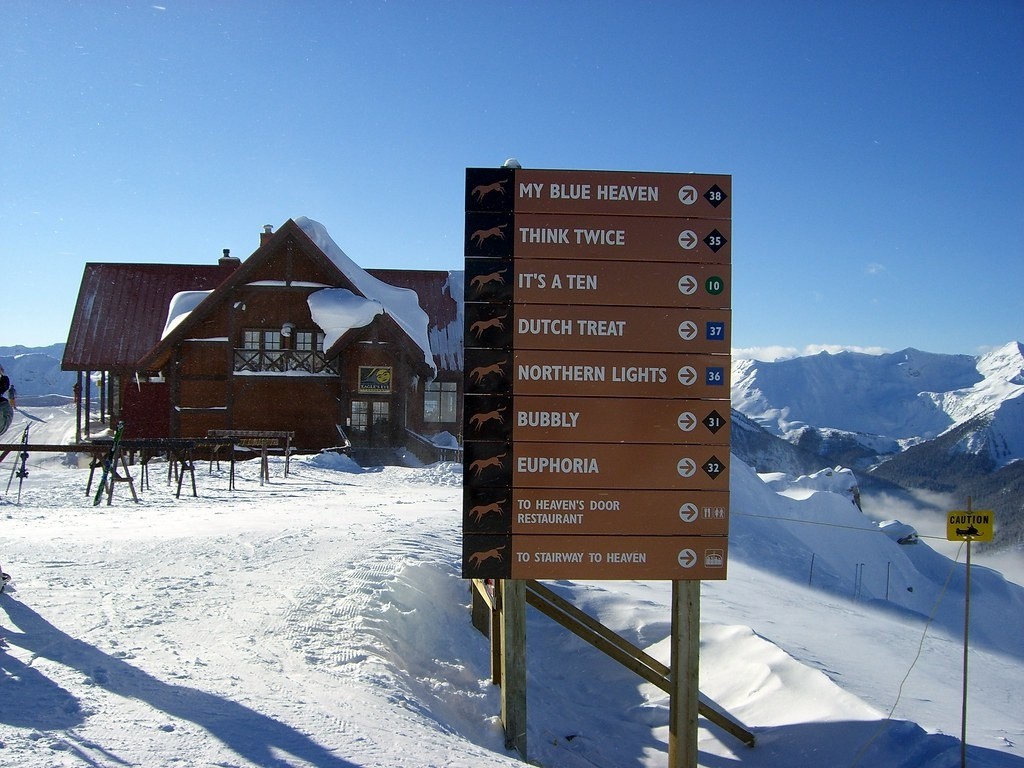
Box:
[92,418,126,506]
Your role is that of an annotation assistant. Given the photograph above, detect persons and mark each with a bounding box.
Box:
[7,384,17,409]
